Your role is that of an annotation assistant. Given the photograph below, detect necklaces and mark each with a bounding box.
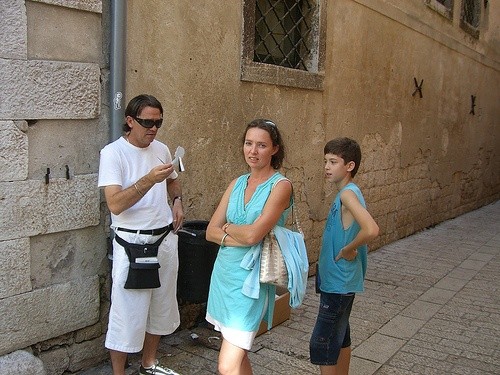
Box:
[125,136,129,142]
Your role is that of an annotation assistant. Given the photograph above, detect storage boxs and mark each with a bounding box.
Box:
[255,286,291,338]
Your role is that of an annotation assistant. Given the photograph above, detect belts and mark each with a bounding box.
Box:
[117,223,173,236]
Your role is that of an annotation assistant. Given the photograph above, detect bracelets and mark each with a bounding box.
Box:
[224,222,231,232]
[133,182,145,198]
[173,196,181,204]
[220,234,228,249]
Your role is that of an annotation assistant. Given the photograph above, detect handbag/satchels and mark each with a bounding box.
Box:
[115,231,170,289]
[259,178,309,296]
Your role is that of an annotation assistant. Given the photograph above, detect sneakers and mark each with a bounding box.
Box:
[138,359,179,375]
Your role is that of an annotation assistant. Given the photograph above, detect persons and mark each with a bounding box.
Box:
[97,94,185,375]
[204,119,294,375]
[309,138,380,375]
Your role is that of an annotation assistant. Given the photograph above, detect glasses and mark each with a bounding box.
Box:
[264,120,277,129]
[134,117,163,129]
